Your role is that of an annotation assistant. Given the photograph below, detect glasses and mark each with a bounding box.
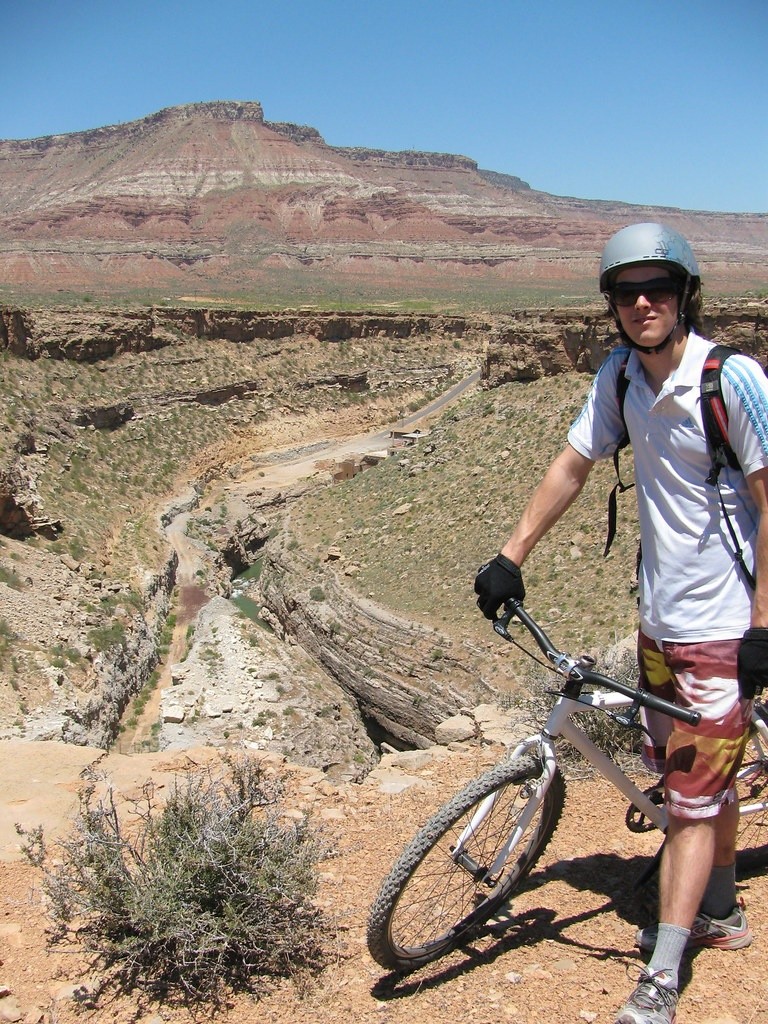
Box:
[605,276,687,307]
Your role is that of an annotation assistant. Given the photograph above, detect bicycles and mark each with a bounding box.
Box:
[363,589,768,971]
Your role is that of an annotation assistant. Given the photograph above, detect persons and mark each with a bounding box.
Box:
[473,222,768,1024]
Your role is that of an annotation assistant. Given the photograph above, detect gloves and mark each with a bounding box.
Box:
[473,553,526,620]
[737,628,768,698]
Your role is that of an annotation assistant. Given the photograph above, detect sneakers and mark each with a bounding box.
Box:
[635,896,753,953]
[614,963,678,1024]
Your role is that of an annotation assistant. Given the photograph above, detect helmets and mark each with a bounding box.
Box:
[598,222,701,293]
